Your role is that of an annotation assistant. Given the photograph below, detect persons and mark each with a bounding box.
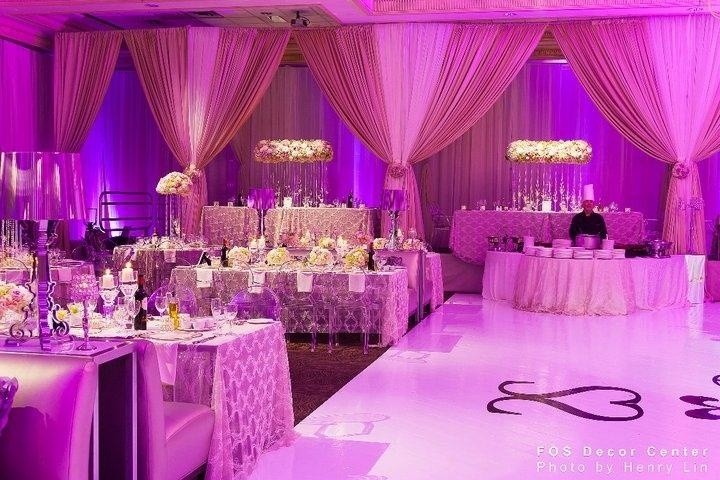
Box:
[107,226,136,252]
[568,184,617,242]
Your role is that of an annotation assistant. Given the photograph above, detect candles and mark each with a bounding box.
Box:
[103,269,113,285]
[121,261,134,282]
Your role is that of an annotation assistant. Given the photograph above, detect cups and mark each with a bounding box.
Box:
[193,321,205,329]
[169,297,180,329]
[214,201,233,207]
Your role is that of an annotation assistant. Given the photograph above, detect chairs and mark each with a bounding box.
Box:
[0,351,97,480]
[287,250,423,322]
[103,340,215,479]
[148,284,282,323]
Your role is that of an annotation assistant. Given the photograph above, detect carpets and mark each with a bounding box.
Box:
[283,291,456,428]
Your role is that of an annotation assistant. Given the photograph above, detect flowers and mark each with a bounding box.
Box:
[155,172,193,196]
[388,160,411,178]
[227,236,419,267]
[252,139,335,164]
[505,140,592,165]
[183,164,200,181]
[672,163,689,178]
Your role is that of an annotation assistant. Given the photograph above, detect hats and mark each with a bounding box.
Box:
[583,184,594,201]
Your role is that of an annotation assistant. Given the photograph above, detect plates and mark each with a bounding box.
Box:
[247,319,273,323]
[523,235,626,260]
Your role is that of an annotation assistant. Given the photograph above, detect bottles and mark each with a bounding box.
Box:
[368,242,375,271]
[151,228,157,244]
[221,238,228,266]
[348,189,352,208]
[135,274,147,330]
[237,189,244,206]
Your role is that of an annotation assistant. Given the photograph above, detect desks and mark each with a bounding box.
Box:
[448,209,646,266]
[1,337,138,479]
[482,254,689,317]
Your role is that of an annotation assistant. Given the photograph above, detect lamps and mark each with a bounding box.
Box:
[0,151,87,346]
[247,187,274,235]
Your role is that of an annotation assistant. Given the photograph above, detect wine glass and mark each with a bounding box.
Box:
[210,297,238,328]
[126,296,141,330]
[155,298,168,329]
